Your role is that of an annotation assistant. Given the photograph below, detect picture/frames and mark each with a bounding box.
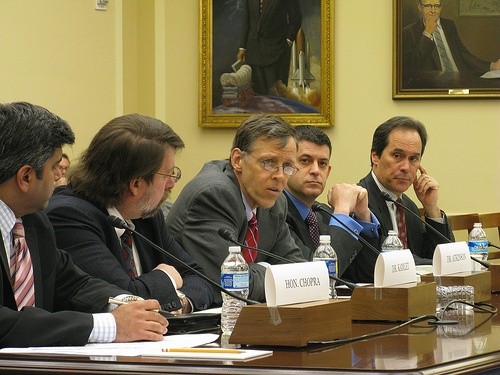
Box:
[391,0,500,101]
[198,0,336,131]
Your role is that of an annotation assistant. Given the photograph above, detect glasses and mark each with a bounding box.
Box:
[248,153,299,175]
[150,167,181,182]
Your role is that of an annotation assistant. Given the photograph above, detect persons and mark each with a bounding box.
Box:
[283,124,380,289]
[0,102,169,347]
[357,116,455,264]
[168,115,307,304]
[47,114,215,313]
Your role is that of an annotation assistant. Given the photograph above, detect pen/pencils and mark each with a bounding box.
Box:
[162,348,246,354]
[108,299,172,315]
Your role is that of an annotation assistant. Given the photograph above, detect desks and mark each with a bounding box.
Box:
[0,290,500,375]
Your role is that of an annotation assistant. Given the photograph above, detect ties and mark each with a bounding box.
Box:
[394,198,408,249]
[10,223,35,311]
[306,212,320,248]
[242,213,259,265]
[120,229,138,279]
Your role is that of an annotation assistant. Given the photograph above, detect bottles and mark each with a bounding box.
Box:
[381,229,404,252]
[313,235,339,299]
[435,285,475,311]
[468,223,489,271]
[219,246,250,334]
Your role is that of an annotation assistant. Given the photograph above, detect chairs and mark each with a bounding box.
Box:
[467,213,500,242]
[447,213,480,234]
